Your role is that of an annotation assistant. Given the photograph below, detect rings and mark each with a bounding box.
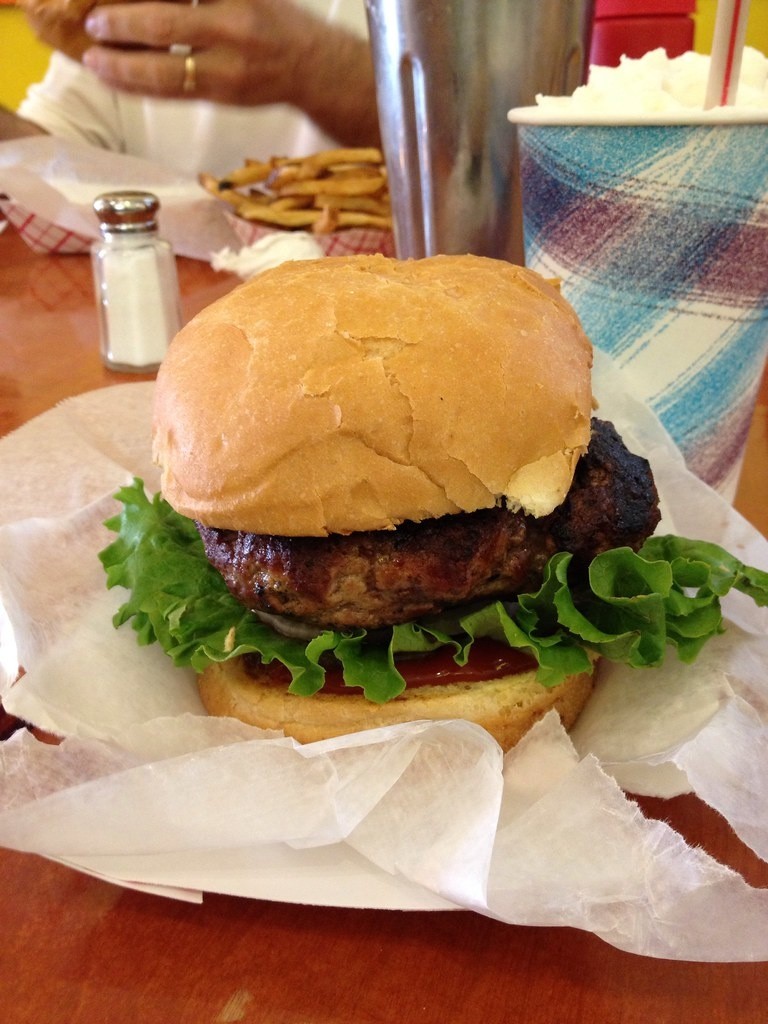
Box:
[183,56,195,93]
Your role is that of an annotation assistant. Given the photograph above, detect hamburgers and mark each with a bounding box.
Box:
[97,256,768,755]
[15,0,193,63]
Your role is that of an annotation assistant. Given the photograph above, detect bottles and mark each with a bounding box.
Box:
[92,189,182,375]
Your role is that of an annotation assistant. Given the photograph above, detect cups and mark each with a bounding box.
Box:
[363,0,588,272]
[583,0,697,70]
[505,106,768,508]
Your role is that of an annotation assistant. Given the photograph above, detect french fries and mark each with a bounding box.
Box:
[197,143,393,233]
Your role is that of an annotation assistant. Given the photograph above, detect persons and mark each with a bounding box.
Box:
[1,1,381,178]
